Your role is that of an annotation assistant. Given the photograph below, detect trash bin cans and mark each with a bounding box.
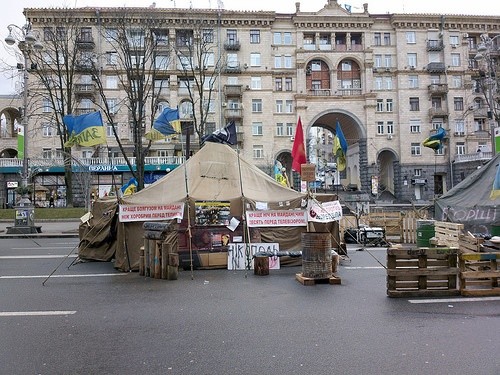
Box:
[301,231,332,280]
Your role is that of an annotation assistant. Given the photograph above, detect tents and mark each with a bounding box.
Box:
[79,142,340,270]
[435,151,500,229]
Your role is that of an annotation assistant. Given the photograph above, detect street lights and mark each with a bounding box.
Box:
[4,24,45,234]
[474,32,497,156]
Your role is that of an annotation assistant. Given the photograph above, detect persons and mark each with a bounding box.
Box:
[49,195,55,208]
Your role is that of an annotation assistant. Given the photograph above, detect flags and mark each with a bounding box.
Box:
[63,111,107,148]
[333,120,348,171]
[423,127,446,151]
[291,120,307,175]
[121,178,138,195]
[201,121,238,148]
[145,107,181,141]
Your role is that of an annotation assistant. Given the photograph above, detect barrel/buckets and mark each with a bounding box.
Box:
[417,224,435,247]
[300,231,333,279]
